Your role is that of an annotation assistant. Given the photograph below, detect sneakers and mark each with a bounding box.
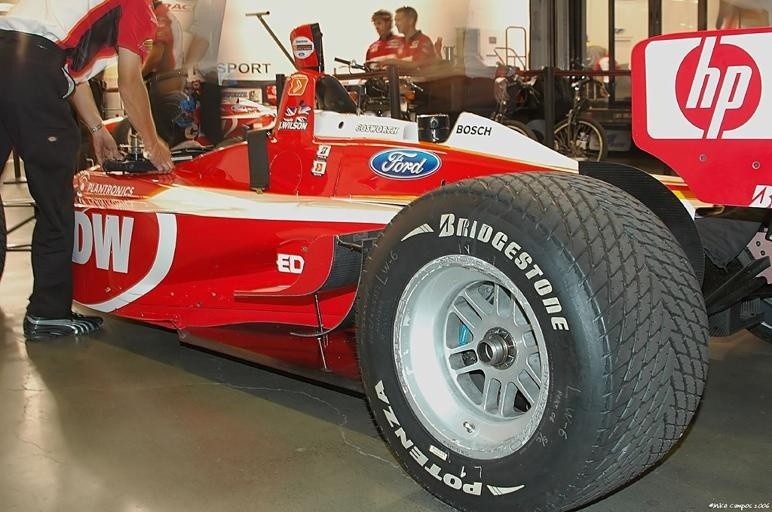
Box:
[22,311,104,342]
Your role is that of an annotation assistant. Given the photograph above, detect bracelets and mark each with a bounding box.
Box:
[90,122,104,135]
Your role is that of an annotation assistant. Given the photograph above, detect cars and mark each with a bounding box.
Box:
[69,24,771,510]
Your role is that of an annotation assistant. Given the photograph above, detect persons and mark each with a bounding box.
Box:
[373,6,436,70]
[364,8,402,60]
[0,1,176,338]
[141,1,189,104]
[182,1,229,147]
